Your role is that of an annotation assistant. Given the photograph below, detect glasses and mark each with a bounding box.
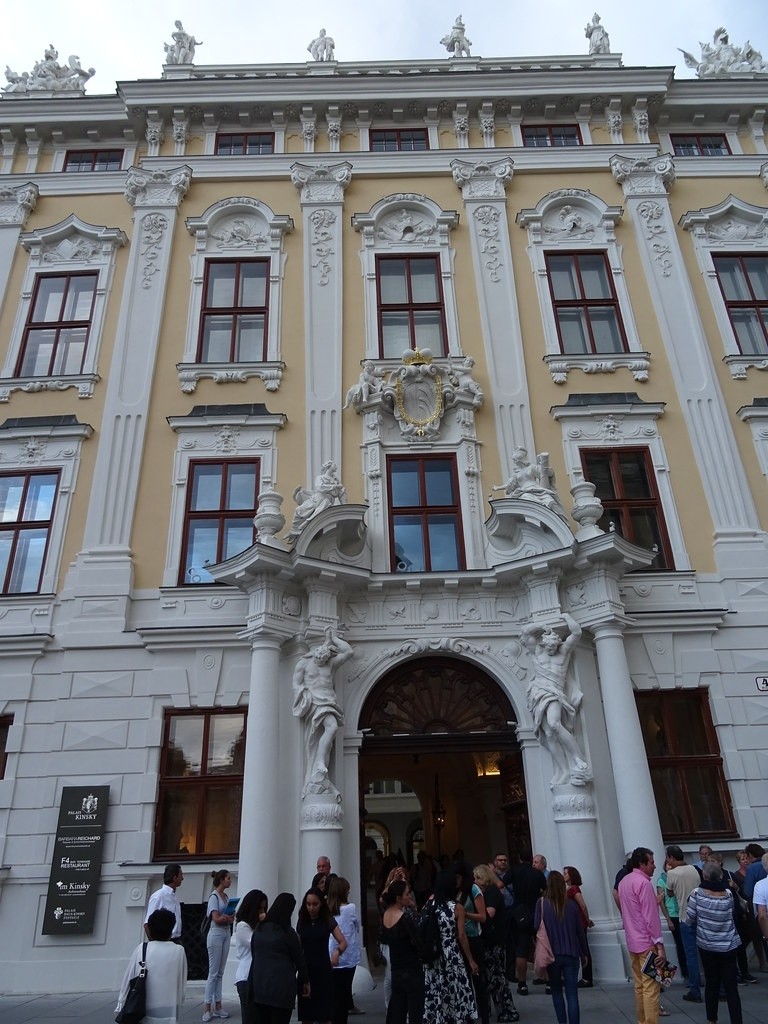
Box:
[496,858,508,862]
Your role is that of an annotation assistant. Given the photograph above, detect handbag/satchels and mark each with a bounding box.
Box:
[466,931,494,953]
[727,870,750,913]
[200,894,219,937]
[114,941,148,1024]
[511,902,533,931]
[533,897,556,982]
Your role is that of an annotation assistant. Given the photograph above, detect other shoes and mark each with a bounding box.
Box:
[374,959,381,967]
[683,991,703,1003]
[700,978,705,987]
[517,985,529,996]
[349,1006,366,1015]
[682,977,689,987]
[544,987,552,994]
[532,977,548,985]
[659,1004,670,1016]
[382,959,387,966]
[578,979,594,988]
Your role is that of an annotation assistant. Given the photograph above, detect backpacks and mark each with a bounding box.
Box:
[414,896,444,964]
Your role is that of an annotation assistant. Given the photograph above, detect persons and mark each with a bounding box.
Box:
[114,864,188,1024]
[518,613,588,787]
[585,12,610,54]
[166,20,203,64]
[282,461,348,545]
[612,844,768,1024]
[684,28,762,76]
[342,360,385,410]
[175,124,184,141]
[447,355,485,396]
[307,29,335,61]
[1,44,96,93]
[305,123,314,139]
[492,446,570,526]
[147,127,159,142]
[291,626,353,800]
[450,15,472,57]
[200,849,595,1024]
[329,123,340,136]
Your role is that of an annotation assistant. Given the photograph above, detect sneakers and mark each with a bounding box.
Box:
[741,972,759,983]
[212,1008,229,1019]
[202,1011,212,1021]
[736,974,748,986]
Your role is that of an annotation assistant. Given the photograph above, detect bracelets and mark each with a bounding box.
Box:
[336,947,342,955]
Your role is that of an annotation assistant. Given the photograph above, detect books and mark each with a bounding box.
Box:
[641,950,678,989]
[224,898,240,915]
